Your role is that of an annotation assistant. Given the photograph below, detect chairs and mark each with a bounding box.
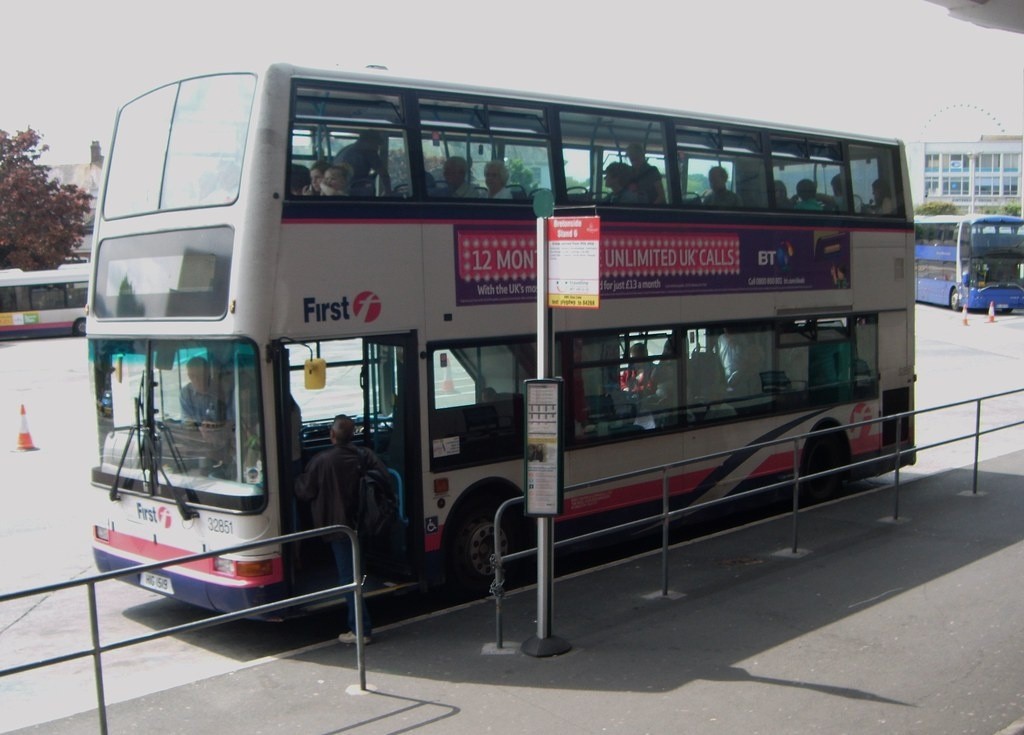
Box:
[393,180,702,207]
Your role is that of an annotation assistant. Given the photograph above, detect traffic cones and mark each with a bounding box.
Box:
[962,304,969,325]
[15,404,38,450]
[985,301,997,323]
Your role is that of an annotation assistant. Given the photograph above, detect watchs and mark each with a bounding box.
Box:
[872,210,876,215]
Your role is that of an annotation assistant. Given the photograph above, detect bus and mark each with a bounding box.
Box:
[913,214,1024,313]
[86,60,918,625]
[0,263,92,340]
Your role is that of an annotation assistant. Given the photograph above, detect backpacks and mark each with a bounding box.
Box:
[353,447,396,535]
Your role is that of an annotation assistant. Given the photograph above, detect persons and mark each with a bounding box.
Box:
[289,393,300,564]
[601,163,638,204]
[866,177,892,215]
[295,414,395,644]
[763,180,790,210]
[703,167,743,208]
[793,179,823,211]
[482,387,497,401]
[443,156,479,197]
[180,356,259,469]
[620,343,656,395]
[623,143,666,205]
[333,130,391,197]
[289,160,353,196]
[484,160,512,198]
[529,444,543,462]
[831,174,861,213]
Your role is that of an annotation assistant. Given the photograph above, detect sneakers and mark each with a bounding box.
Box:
[338,632,372,644]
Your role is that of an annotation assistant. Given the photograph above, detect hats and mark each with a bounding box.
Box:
[602,161,632,178]
